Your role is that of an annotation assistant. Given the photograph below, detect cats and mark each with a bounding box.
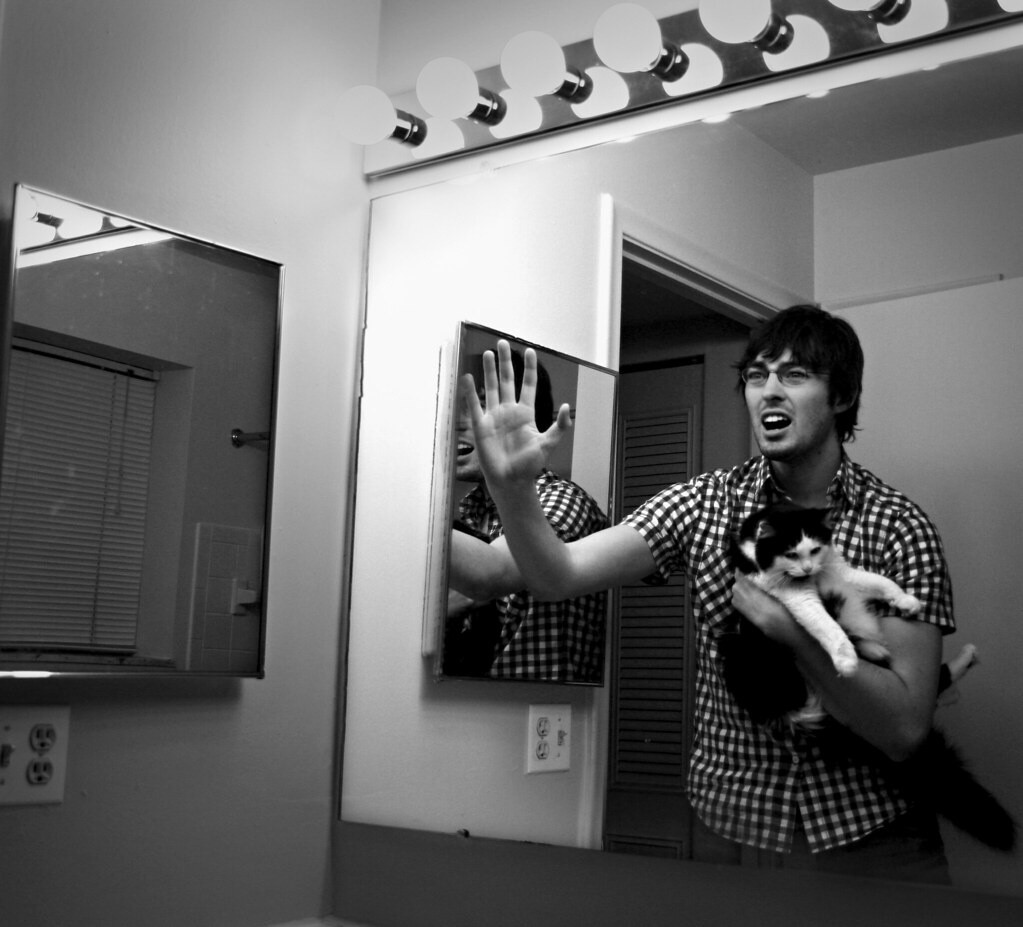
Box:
[444,516,501,677]
[714,502,1023,855]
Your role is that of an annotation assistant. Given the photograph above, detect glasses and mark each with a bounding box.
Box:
[740,365,829,386]
[456,399,486,417]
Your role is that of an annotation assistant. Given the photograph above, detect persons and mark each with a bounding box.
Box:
[461,301,953,887]
[443,343,608,685]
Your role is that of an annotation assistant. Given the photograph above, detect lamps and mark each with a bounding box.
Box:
[336,0,1023,153]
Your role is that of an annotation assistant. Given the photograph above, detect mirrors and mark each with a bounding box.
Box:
[2,185,284,683]
[428,320,622,688]
[331,15,1023,899]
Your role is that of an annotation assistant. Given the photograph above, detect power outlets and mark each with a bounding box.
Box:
[0,704,72,808]
[525,703,572,775]
